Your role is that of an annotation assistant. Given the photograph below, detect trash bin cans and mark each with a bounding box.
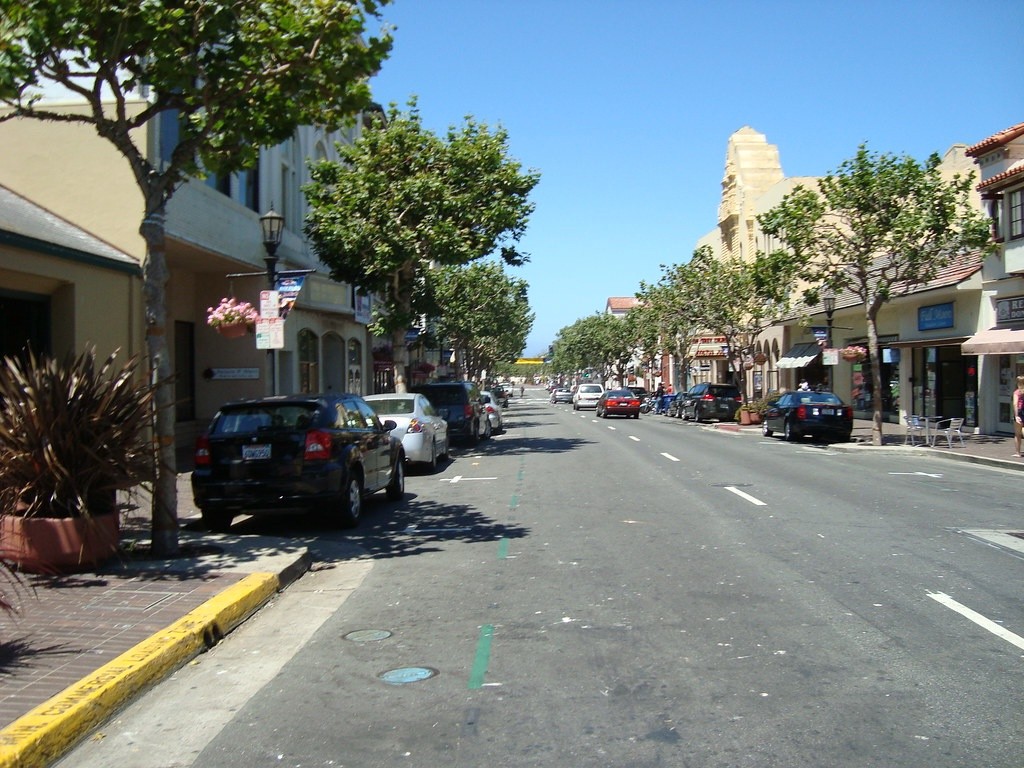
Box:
[750,411,759,422]
[741,408,750,424]
[663,395,675,415]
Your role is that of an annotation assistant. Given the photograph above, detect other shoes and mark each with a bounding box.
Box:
[1012,453,1020,457]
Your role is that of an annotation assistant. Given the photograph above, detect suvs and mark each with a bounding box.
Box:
[573,383,605,411]
[191,392,406,529]
[640,382,743,422]
[409,381,513,445]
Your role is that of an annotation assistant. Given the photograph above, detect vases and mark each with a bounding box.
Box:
[842,355,859,362]
[0,513,121,572]
[216,324,249,338]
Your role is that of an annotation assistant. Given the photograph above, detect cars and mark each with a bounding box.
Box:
[596,386,650,419]
[761,390,853,442]
[362,392,449,471]
[546,380,573,405]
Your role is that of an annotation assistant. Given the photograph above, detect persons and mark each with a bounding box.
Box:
[653,382,672,414]
[521,387,524,397]
[798,379,811,391]
[1011,375,1024,457]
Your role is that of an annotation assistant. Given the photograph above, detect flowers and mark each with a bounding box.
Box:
[838,346,867,357]
[370,342,393,356]
[419,360,437,371]
[206,298,258,327]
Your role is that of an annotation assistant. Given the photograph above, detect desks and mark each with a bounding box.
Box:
[912,415,942,448]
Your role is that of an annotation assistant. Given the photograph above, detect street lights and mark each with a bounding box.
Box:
[258,200,287,397]
[821,289,839,390]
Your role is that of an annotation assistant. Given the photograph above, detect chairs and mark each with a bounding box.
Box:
[237,416,264,433]
[932,418,965,448]
[903,415,925,447]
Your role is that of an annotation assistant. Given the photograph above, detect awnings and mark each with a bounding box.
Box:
[961,325,1024,355]
[774,343,822,368]
[888,336,973,347]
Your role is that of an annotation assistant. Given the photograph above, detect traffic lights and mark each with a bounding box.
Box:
[584,372,589,378]
